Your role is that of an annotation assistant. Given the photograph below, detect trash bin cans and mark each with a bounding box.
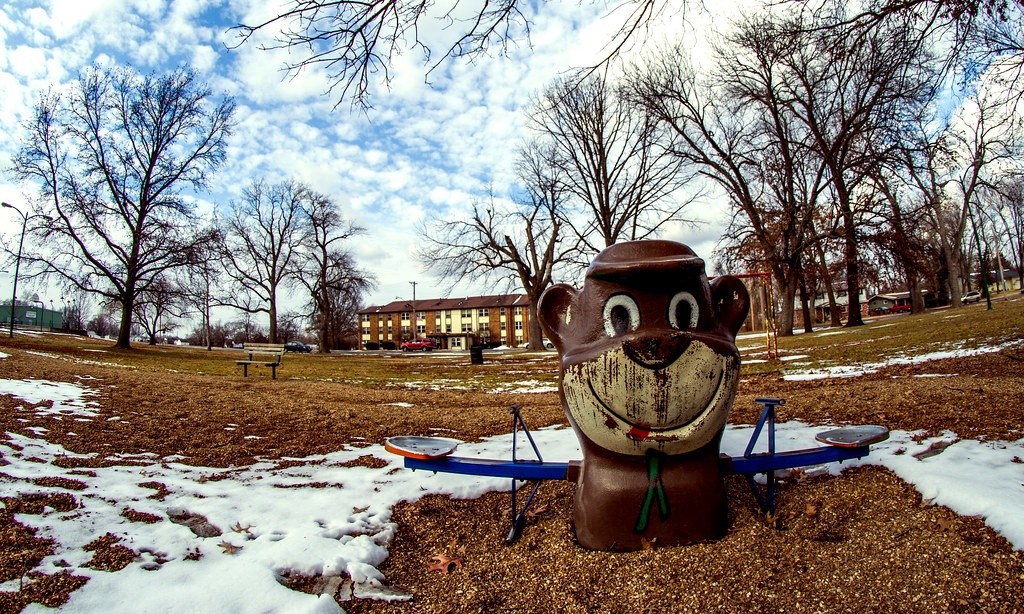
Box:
[469,346,484,365]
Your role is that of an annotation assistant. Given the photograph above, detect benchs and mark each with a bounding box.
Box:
[235,340,288,380]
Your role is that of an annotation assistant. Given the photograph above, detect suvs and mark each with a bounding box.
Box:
[960,291,982,304]
[401,338,434,352]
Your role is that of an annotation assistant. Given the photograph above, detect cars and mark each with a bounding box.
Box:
[523,337,554,348]
[868,302,910,314]
[284,341,312,353]
